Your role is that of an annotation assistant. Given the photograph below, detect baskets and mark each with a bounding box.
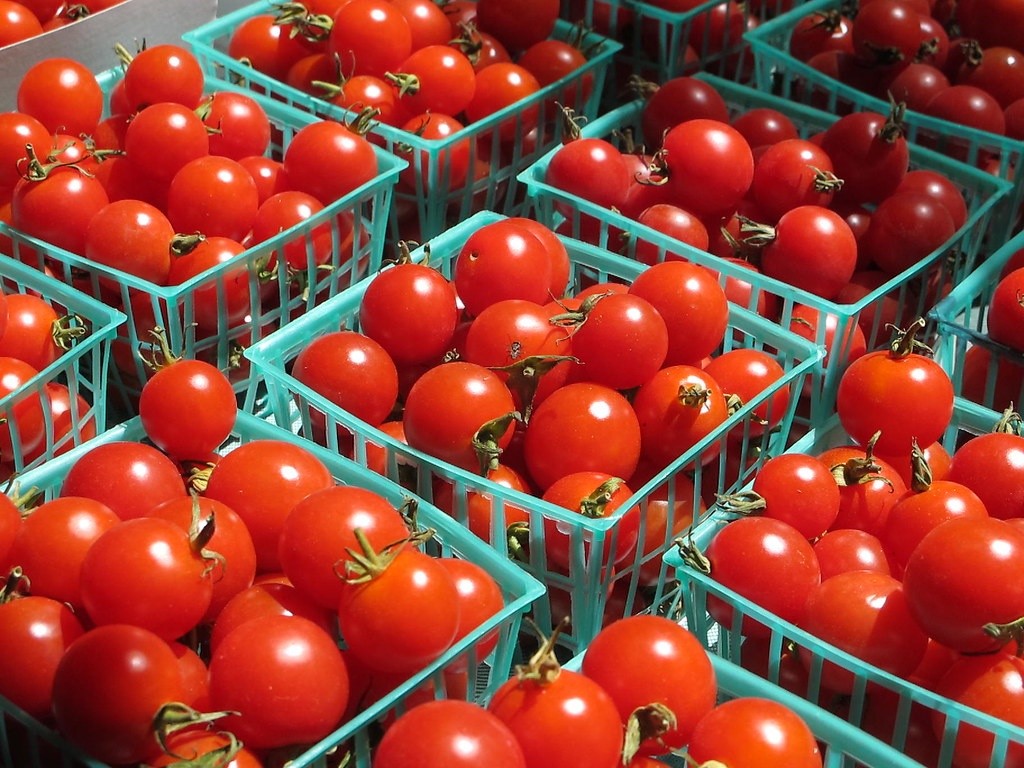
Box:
[0,0,1024,768]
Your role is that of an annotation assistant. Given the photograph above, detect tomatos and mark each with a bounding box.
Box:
[0,0,1024,768]
